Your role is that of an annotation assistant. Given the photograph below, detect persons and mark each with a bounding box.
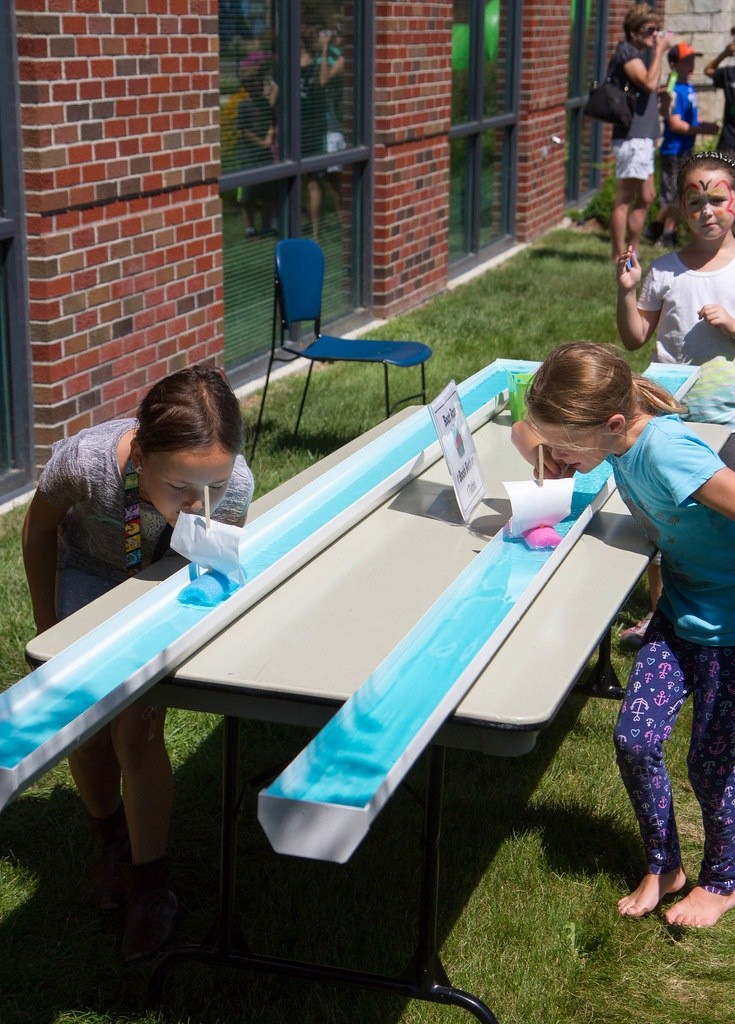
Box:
[704,25,735,151]
[512,339,735,928]
[23,364,255,962]
[606,2,672,264]
[615,151,735,471]
[647,42,720,247]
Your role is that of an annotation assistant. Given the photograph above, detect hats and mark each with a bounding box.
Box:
[667,41,703,61]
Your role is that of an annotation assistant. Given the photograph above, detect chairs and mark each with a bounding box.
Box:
[247,238,430,469]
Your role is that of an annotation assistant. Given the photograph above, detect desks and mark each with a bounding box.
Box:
[24,404,735,1024]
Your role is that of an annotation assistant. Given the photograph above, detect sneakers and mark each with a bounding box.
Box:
[647,221,683,253]
[91,822,131,912]
[120,866,178,960]
[245,224,278,240]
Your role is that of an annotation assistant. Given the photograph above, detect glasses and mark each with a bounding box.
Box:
[640,25,662,36]
